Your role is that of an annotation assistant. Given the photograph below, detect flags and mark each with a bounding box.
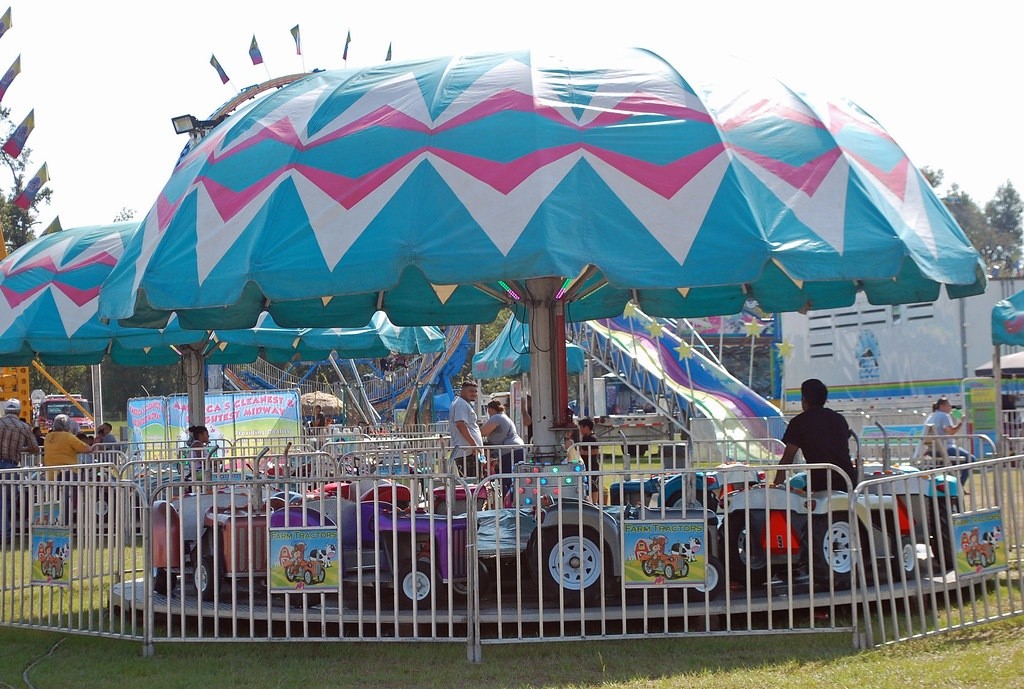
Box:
[206,22,393,82]
[0,7,64,235]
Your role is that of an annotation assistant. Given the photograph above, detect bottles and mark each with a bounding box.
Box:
[477,451,487,464]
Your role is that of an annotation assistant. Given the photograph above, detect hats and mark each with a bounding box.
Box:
[3,398,21,411]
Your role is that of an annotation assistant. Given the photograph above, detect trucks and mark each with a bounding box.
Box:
[28,393,97,437]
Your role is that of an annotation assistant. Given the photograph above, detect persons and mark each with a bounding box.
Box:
[45,413,96,542]
[0,397,40,548]
[925,397,977,495]
[19,406,119,471]
[186,426,223,473]
[449,382,486,477]
[310,405,326,427]
[480,401,526,500]
[774,378,859,493]
[578,419,603,505]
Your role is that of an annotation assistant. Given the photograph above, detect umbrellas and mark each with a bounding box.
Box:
[0,49,759,477]
